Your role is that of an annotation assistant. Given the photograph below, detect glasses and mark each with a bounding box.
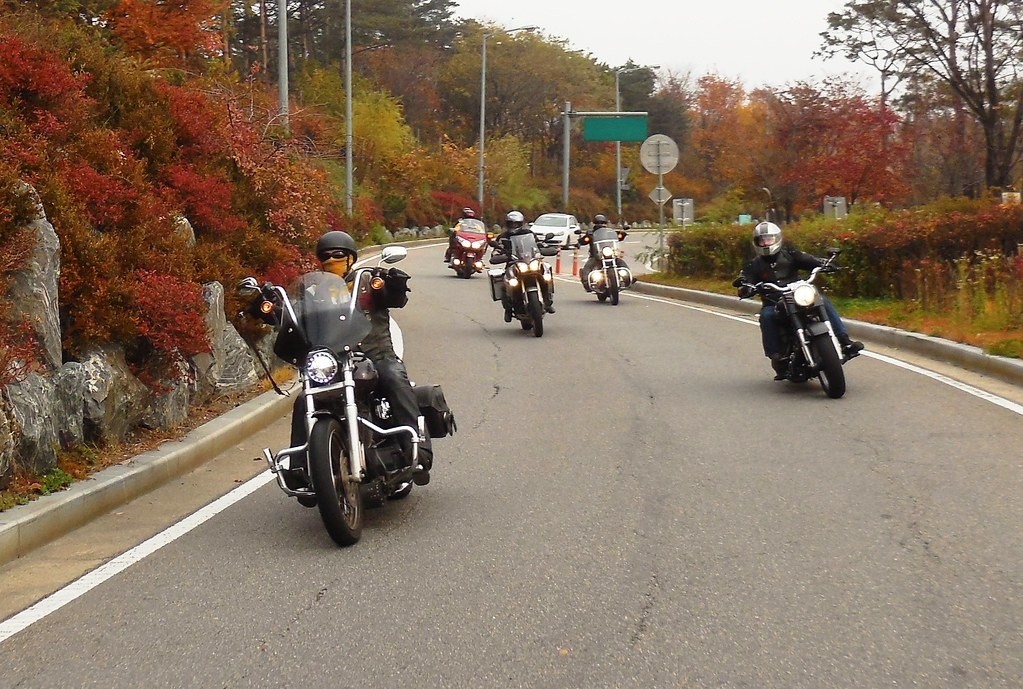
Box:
[316,251,347,261]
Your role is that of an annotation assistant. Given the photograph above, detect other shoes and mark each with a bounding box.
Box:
[443,258,449,262]
[625,278,638,285]
[277,470,317,508]
[544,305,554,313]
[401,446,430,486]
[772,354,788,376]
[504,310,512,323]
[841,341,864,358]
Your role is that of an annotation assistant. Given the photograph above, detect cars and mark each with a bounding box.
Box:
[528,212,582,250]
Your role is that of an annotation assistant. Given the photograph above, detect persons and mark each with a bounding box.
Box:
[491,211,560,322]
[738,222,864,374]
[443,207,481,263]
[577,215,637,292]
[247,231,432,507]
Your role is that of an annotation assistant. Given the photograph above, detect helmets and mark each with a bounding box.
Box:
[752,222,782,256]
[465,210,474,216]
[504,210,524,233]
[592,214,607,224]
[315,231,357,262]
[463,207,470,214]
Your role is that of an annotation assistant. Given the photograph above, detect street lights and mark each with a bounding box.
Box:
[477,24,541,222]
[614,63,661,229]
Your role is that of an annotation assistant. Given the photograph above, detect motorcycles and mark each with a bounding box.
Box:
[732,245,861,399]
[234,244,459,546]
[574,224,633,306]
[443,218,495,281]
[487,228,556,338]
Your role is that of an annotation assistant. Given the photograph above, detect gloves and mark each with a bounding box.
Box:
[380,267,405,293]
[736,286,753,297]
[820,260,837,273]
